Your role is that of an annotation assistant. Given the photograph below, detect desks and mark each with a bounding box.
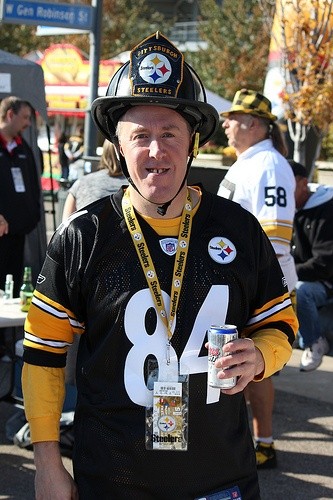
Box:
[0,298,29,405]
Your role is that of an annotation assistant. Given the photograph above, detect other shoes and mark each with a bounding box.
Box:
[254,442,278,468]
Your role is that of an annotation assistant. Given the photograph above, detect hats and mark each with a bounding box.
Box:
[286,160,308,177]
[219,88,278,122]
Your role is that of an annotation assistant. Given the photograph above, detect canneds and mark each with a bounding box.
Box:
[208,325,239,390]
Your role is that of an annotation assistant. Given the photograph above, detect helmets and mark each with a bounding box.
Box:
[90,29,221,150]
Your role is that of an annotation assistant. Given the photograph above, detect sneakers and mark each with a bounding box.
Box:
[298,336,332,373]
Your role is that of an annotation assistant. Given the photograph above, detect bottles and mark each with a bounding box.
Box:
[20,267,34,312]
[5,275,14,299]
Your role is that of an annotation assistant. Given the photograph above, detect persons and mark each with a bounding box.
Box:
[20,95,300,498]
[284,160,333,372]
[61,137,131,223]
[34,111,88,188]
[0,96,42,299]
[215,88,297,470]
